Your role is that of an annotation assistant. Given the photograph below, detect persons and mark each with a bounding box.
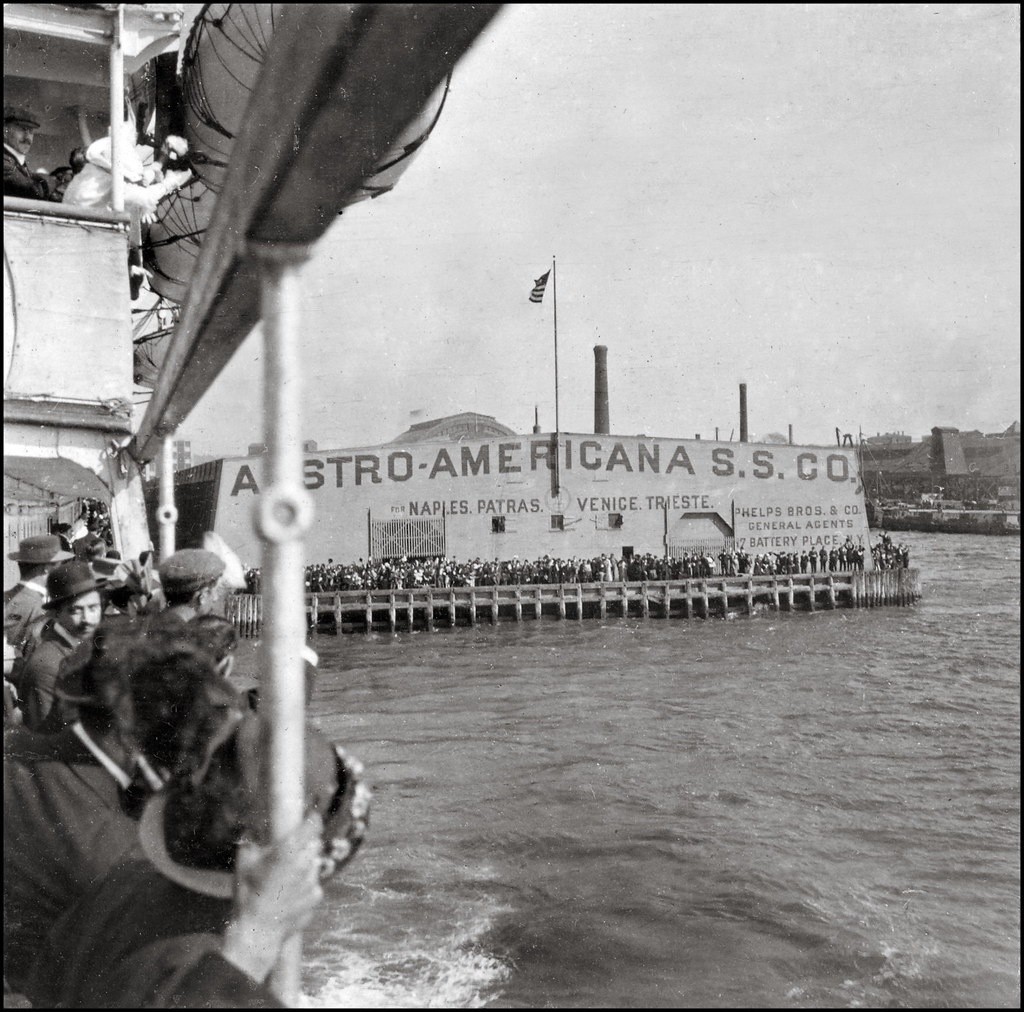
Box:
[5,496,371,1008]
[242,531,911,594]
[62,133,193,300]
[3,106,76,200]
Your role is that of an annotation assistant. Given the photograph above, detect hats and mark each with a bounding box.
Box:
[41,560,110,609]
[8,535,76,563]
[88,557,127,590]
[159,548,226,593]
[138,711,373,898]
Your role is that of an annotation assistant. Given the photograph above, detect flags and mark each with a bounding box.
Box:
[529,271,551,304]
